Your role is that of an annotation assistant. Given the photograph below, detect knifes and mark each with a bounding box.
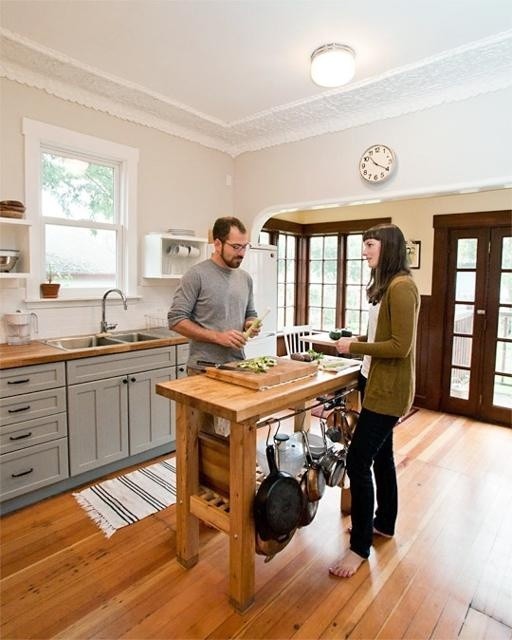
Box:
[197,359,249,371]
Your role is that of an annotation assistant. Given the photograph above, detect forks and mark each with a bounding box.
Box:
[1,200,25,207]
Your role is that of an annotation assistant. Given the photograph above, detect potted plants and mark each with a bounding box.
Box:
[40,264,61,298]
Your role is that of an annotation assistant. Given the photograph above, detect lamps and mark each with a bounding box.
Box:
[310,42,356,88]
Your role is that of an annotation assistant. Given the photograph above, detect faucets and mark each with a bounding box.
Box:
[100,288,128,332]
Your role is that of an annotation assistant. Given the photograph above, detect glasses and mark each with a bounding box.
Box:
[223,241,251,253]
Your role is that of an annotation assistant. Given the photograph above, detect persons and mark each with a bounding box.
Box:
[329,224,422,577]
[168,215,264,437]
[404,245,416,265]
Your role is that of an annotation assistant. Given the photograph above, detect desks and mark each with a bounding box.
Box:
[156,349,364,615]
[299,332,360,356]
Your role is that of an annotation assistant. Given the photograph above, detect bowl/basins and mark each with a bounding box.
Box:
[0,249,21,272]
[0,205,27,219]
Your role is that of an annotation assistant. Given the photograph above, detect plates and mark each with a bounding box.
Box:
[167,228,196,237]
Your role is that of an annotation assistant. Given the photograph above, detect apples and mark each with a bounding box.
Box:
[342,329,352,337]
[329,329,341,340]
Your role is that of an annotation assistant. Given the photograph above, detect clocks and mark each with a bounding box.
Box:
[359,144,396,184]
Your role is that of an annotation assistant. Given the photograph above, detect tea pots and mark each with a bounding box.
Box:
[5,309,41,345]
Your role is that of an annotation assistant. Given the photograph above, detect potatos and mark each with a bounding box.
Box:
[291,353,303,361]
[302,353,313,362]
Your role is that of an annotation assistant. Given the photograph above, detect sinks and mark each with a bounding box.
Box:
[106,332,163,349]
[43,333,124,351]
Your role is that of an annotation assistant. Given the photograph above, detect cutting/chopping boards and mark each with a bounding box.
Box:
[205,356,319,388]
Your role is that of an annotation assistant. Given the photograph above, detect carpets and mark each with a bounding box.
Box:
[71,456,176,540]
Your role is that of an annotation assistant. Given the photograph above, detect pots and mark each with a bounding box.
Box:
[252,397,360,562]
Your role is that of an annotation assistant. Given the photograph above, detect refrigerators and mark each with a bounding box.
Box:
[208,244,278,359]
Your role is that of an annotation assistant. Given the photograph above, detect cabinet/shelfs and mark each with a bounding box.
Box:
[142,233,208,279]
[0,342,191,516]
[0,216,33,279]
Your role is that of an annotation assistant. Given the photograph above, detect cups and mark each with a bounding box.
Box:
[207,229,213,243]
[166,241,201,257]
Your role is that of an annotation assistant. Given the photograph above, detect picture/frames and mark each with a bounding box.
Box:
[405,240,421,269]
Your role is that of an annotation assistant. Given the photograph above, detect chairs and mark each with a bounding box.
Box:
[282,325,312,355]
